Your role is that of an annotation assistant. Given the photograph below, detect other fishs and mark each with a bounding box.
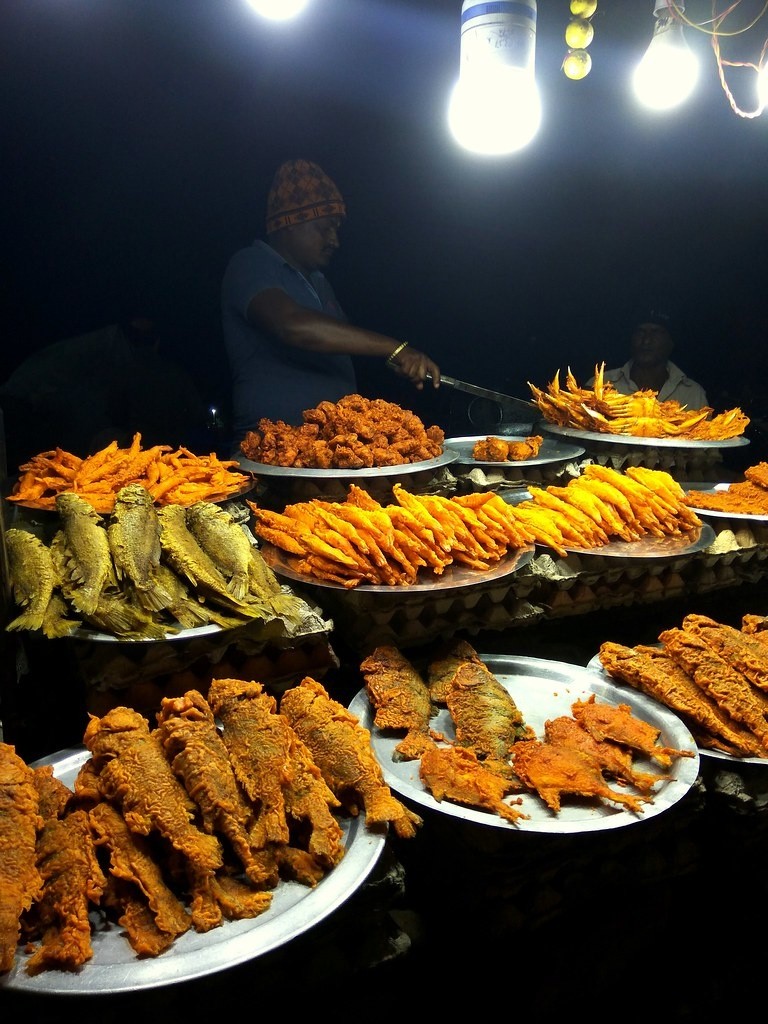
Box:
[0,432,768,984]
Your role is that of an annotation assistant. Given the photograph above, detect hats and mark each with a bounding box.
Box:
[633,295,680,340]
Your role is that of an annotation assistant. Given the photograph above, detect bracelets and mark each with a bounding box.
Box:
[385,341,408,365]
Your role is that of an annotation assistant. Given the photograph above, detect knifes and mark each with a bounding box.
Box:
[386,361,544,415]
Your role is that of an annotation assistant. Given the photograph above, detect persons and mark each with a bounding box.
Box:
[584,306,726,467]
[221,160,442,460]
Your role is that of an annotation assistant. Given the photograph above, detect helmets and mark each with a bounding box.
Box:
[264,159,344,233]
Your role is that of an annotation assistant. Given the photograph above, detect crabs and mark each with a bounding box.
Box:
[527,359,751,439]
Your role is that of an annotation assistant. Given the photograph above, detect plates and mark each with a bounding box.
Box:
[347,654,700,837]
[259,539,536,593]
[3,746,389,994]
[40,575,298,644]
[496,488,714,557]
[441,435,585,467]
[537,415,753,447]
[674,480,768,525]
[585,642,768,764]
[229,446,462,478]
[9,463,263,508]
